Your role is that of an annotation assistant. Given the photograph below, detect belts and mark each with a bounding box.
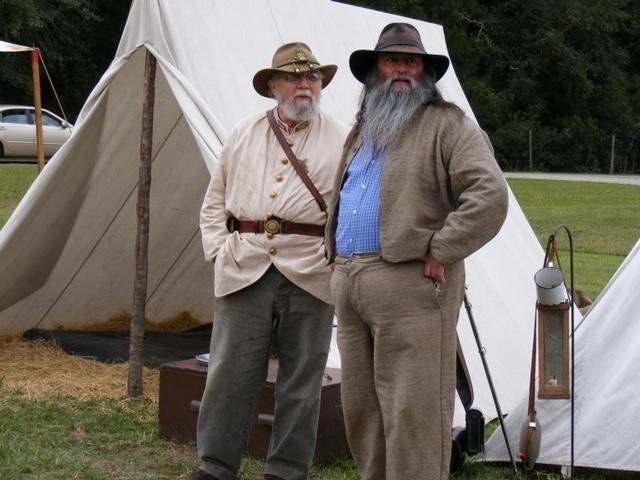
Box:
[234,216,324,237]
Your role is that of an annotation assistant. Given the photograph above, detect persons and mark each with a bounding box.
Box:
[178,41,355,480]
[323,21,510,480]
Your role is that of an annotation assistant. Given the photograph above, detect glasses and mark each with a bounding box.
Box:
[275,73,320,82]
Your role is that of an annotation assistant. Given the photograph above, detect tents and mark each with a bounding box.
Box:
[469,236,640,471]
[0,0,584,433]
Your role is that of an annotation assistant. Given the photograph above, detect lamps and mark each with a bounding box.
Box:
[537,225,574,480]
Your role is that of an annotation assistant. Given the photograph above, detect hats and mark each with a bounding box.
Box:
[349,22,449,85]
[253,41,338,98]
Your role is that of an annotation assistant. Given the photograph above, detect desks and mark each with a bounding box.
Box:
[160,356,352,463]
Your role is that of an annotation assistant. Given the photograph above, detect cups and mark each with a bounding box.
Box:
[534,262,569,305]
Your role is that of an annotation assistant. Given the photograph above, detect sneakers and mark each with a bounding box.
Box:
[184,470,216,480]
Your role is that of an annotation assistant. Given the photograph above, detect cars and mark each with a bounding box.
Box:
[0,105,74,158]
